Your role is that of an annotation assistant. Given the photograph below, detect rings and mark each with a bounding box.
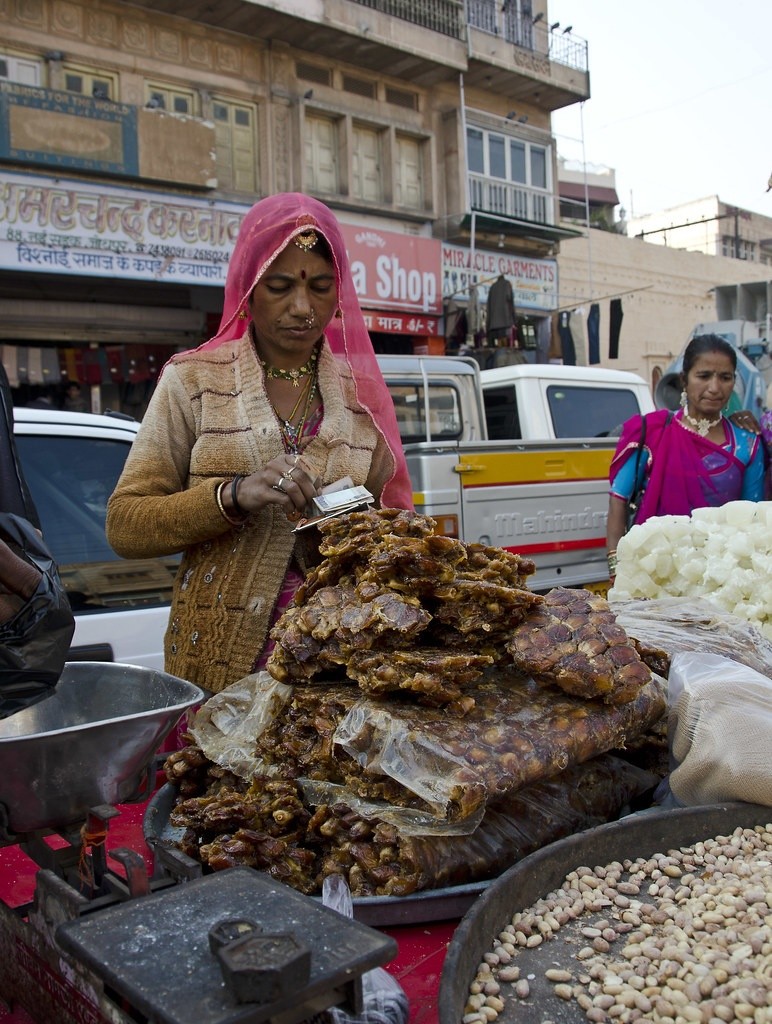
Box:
[282,465,296,481]
[294,455,302,465]
[744,415,750,420]
[738,416,742,421]
[273,477,285,493]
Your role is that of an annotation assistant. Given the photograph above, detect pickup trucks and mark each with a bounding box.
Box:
[367,348,632,600]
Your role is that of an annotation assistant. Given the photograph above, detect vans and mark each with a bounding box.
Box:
[478,360,658,440]
[12,401,185,677]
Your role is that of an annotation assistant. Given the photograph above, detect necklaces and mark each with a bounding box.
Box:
[684,405,723,438]
[256,349,318,453]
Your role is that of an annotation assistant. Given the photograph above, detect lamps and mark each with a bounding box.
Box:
[500,0,574,36]
[505,111,528,128]
[48,53,67,69]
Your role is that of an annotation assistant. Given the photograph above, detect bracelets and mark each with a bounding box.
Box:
[606,550,617,577]
[216,479,247,525]
[231,474,246,516]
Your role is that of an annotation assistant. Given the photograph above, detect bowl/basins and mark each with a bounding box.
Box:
[0,661,204,842]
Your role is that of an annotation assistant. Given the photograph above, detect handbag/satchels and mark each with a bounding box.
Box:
[0,387,76,720]
[625,410,673,533]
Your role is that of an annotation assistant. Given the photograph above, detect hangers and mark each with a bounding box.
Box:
[443,271,518,306]
[463,342,527,361]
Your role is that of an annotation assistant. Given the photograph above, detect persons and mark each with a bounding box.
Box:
[605,333,772,588]
[108,191,412,703]
[0,361,76,722]
